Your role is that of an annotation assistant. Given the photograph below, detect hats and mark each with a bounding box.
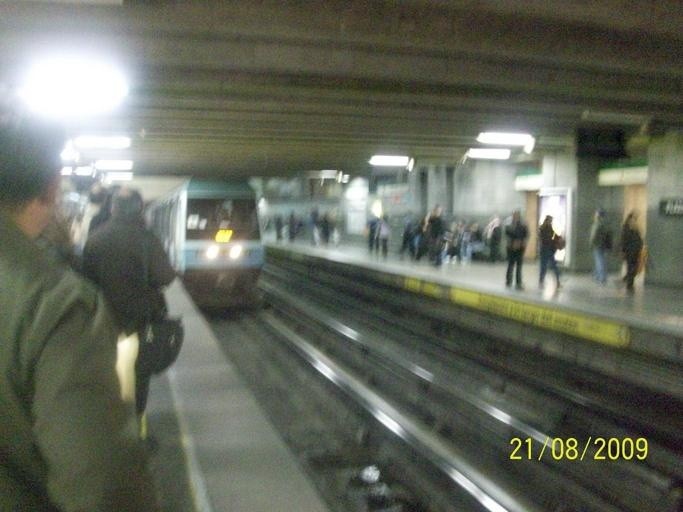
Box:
[596,206,606,217]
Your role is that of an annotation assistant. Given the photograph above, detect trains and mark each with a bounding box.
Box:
[143,171,267,311]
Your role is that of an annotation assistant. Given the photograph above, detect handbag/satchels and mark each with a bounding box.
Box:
[555,236,564,249]
[145,320,184,372]
[511,238,520,252]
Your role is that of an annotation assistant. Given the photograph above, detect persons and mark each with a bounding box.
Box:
[589,208,643,296]
[33,179,177,439]
[271,206,333,249]
[366,202,447,268]
[537,214,564,289]
[448,212,502,262]
[0,108,160,512]
[503,208,530,290]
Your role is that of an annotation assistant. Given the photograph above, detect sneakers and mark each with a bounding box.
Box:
[137,410,147,440]
[507,280,523,289]
[538,280,563,291]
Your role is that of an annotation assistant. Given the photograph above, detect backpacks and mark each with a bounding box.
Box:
[598,217,612,250]
